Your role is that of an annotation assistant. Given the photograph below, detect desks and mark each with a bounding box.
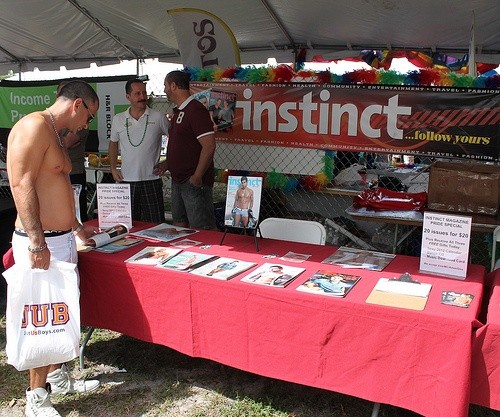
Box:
[345,196,497,264]
[84,155,171,216]
[2,217,486,417]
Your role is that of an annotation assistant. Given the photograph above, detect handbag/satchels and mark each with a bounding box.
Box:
[2,260,79,372]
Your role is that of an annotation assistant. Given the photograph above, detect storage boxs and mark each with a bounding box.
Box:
[427,160,500,224]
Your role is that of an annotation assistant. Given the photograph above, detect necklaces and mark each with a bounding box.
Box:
[47,107,64,147]
[126,116,149,147]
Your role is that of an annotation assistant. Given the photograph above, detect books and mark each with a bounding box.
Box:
[240,262,306,288]
[88,223,144,254]
[124,246,183,266]
[134,222,199,242]
[155,251,220,273]
[189,257,257,280]
[224,176,262,230]
[296,270,361,297]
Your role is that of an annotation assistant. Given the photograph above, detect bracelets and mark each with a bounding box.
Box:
[74,224,82,234]
[28,243,47,254]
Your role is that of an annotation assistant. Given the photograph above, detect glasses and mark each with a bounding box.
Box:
[75,96,95,123]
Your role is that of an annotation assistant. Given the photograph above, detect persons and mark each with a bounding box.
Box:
[197,95,234,132]
[56,81,89,225]
[206,261,239,276]
[250,265,292,285]
[106,225,124,238]
[7,81,101,417]
[304,273,346,294]
[185,255,196,263]
[152,249,168,260]
[109,79,169,223]
[165,70,216,229]
[232,177,253,228]
[143,228,195,239]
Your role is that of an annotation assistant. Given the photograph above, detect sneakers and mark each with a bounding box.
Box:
[25,382,62,417]
[45,363,101,397]
